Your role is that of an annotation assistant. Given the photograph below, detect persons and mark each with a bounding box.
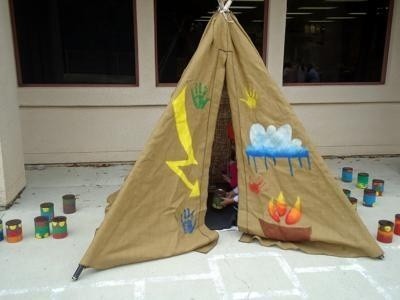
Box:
[221,122,238,188]
[220,186,239,207]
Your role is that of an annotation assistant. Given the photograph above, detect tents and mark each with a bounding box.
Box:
[71,0,384,280]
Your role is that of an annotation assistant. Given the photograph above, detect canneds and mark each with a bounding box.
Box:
[41,203,54,222]
[5,219,23,243]
[52,216,67,239]
[0,219,4,241]
[377,220,394,243]
[212,189,227,209]
[394,214,400,236]
[63,194,75,214]
[342,167,384,212]
[34,216,50,238]
